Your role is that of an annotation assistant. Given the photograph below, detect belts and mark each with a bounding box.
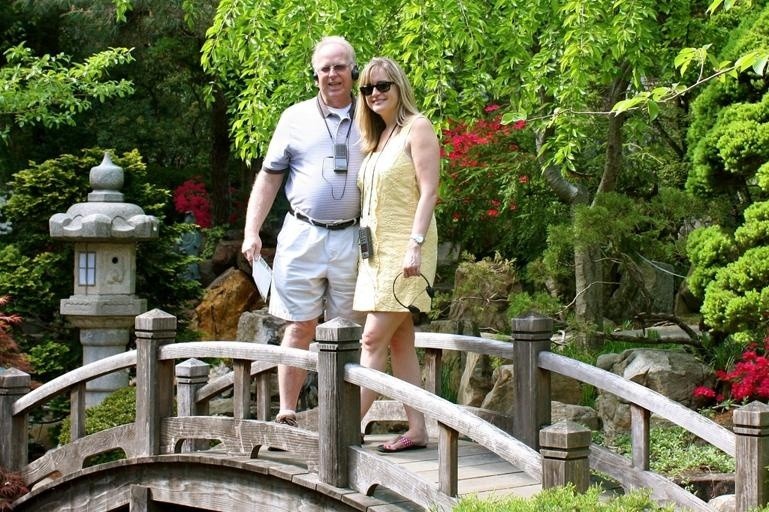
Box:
[288,207,360,230]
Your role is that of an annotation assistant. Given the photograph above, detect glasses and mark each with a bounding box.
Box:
[360,80,395,96]
[321,62,353,72]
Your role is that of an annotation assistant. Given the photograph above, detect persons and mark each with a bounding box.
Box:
[355,58,441,455]
[241,35,365,452]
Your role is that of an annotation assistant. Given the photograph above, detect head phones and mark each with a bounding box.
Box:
[393,271,435,313]
[313,65,359,80]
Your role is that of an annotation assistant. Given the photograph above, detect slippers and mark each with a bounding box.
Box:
[376,434,427,453]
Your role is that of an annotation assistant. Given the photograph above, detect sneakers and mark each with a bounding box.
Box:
[268,415,298,452]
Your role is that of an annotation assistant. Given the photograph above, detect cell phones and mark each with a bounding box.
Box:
[333,143,348,172]
[358,227,373,259]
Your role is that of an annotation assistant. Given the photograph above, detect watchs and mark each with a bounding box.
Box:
[410,234,426,245]
